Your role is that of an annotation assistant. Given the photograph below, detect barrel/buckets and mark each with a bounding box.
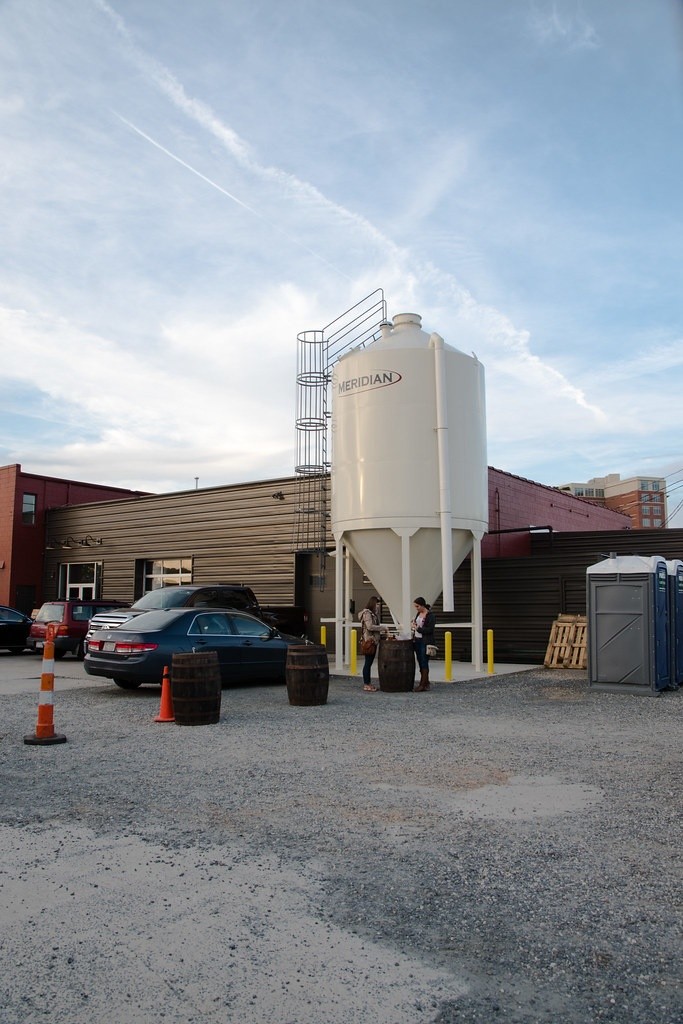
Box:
[171,652,221,726]
[378,640,415,693]
[286,644,330,706]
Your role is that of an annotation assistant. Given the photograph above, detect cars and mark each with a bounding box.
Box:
[80,607,315,690]
[0,606,37,656]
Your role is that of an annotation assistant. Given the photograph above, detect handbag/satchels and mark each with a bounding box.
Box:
[360,637,375,653]
[426,645,439,656]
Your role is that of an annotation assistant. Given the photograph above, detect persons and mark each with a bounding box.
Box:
[412,597,436,692]
[357,596,388,692]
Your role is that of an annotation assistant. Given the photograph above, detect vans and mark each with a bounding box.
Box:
[26,596,132,660]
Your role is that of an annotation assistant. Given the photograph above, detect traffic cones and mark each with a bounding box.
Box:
[154,665,175,723]
[23,622,68,746]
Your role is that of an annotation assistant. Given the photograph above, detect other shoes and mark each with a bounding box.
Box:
[363,684,377,691]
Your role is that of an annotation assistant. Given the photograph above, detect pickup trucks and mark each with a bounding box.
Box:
[84,582,315,654]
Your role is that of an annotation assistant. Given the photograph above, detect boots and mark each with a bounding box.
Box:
[415,669,431,692]
